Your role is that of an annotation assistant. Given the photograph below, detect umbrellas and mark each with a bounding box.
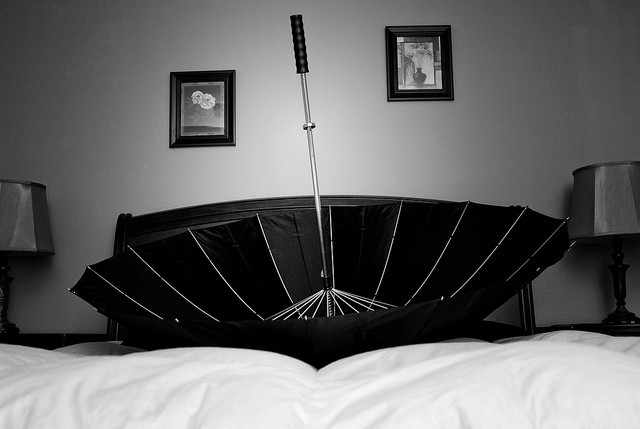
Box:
[66,14,577,368]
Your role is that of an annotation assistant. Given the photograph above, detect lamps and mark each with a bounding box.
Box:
[568,160,639,324]
[1,178,56,344]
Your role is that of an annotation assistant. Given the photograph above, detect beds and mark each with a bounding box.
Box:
[0,195,639,429]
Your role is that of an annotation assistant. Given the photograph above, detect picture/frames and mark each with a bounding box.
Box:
[384,24,453,103]
[169,69,236,148]
